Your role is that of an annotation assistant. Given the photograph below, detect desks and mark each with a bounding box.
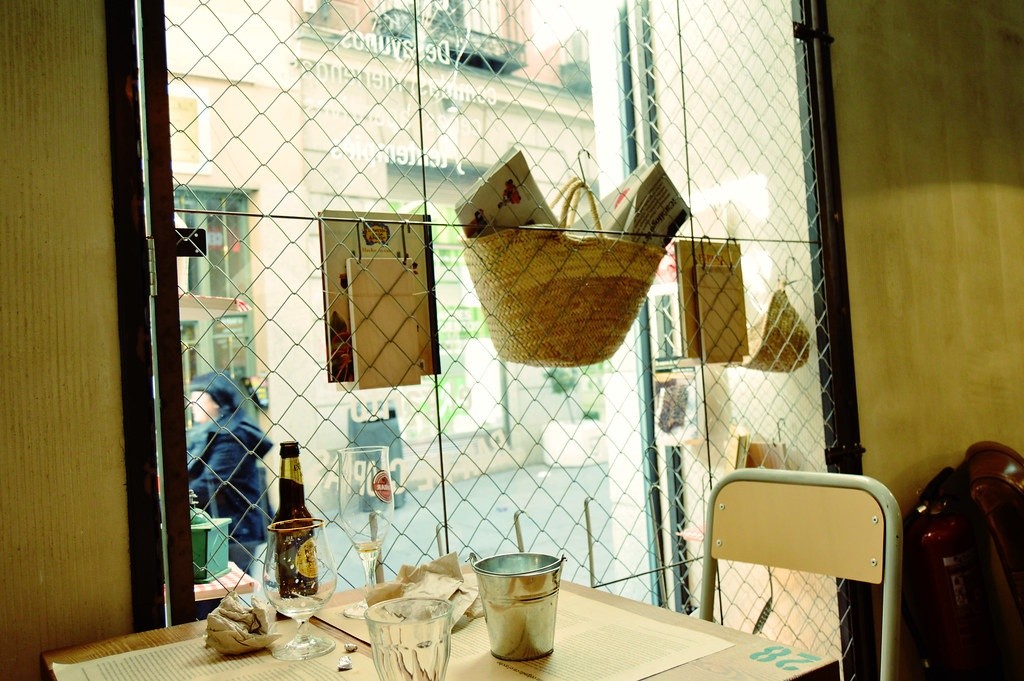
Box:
[41,564,840,681]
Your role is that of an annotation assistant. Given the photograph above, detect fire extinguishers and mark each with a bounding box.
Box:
[901,463,1004,681]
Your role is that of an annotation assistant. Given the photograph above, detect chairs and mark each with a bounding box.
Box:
[699,468,903,681]
[228,467,269,567]
[966,441,1024,627]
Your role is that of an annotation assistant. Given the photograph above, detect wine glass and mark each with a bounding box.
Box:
[335,445,395,620]
[262,517,337,662]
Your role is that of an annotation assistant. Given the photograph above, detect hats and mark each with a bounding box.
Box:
[740,290,809,372]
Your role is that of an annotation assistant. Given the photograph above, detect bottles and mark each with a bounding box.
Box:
[276,440,320,615]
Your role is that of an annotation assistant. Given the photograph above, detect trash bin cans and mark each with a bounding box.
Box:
[347,398,407,513]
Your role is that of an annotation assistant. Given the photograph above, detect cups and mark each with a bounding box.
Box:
[364,597,455,681]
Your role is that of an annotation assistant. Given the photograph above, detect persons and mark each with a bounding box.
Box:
[158,370,274,576]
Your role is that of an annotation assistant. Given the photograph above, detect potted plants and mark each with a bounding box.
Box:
[542,367,608,467]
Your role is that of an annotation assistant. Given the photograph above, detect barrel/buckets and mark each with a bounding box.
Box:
[464,552,568,661]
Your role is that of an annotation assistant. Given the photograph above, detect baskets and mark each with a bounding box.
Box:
[465,177,667,367]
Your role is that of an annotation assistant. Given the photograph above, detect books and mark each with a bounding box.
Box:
[605,161,691,247]
